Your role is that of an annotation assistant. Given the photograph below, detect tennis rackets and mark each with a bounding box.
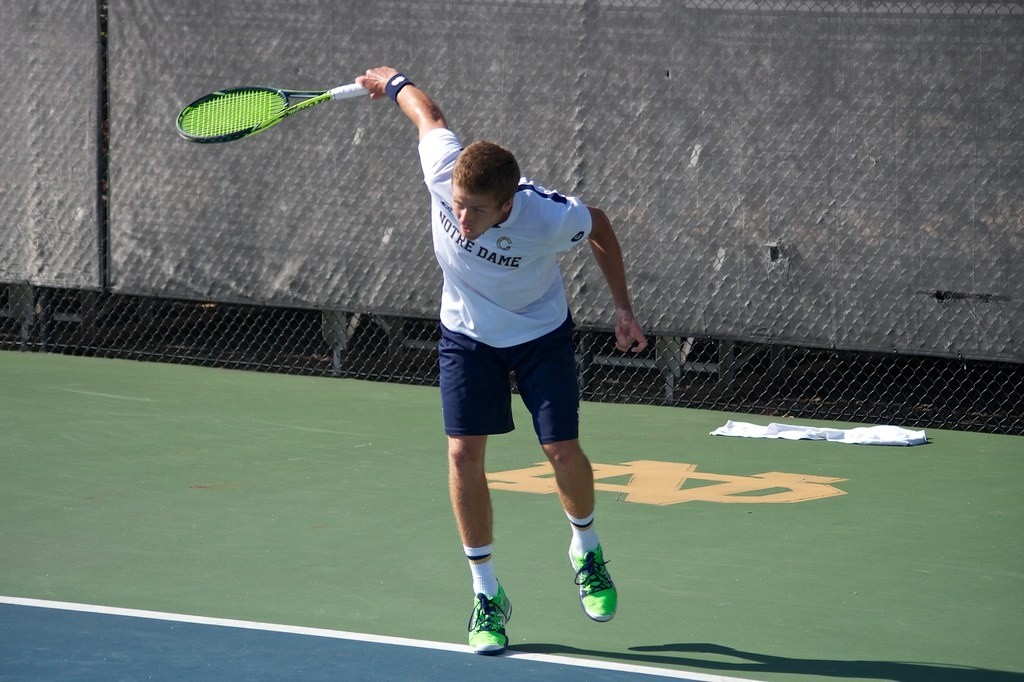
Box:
[175,83,369,145]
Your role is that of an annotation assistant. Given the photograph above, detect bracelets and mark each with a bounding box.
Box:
[385,73,414,102]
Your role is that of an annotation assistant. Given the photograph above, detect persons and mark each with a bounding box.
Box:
[356,66,648,655]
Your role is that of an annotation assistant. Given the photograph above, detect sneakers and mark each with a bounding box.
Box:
[568,542,618,622]
[468,577,513,655]
